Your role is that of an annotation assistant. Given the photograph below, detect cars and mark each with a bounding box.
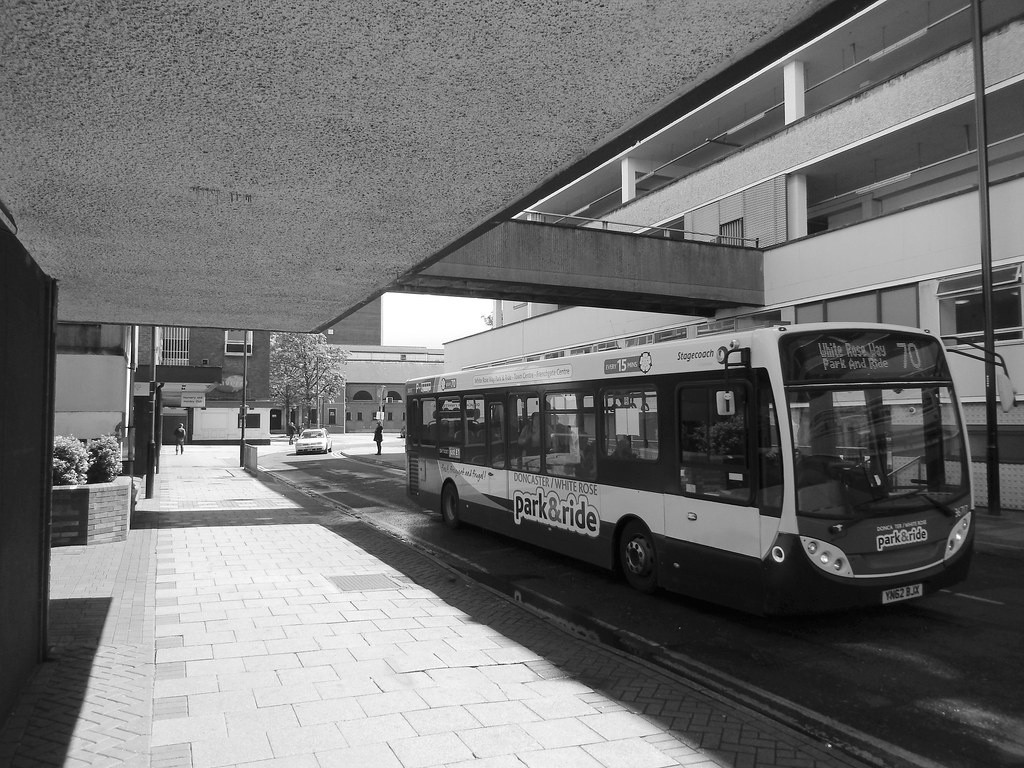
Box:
[293,427,333,455]
[399,425,406,438]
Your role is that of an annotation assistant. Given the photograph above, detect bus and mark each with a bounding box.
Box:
[398,321,1018,620]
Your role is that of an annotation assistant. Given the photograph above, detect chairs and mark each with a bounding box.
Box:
[426,419,520,454]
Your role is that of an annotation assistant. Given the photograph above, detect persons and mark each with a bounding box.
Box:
[747,446,779,469]
[174,423,186,455]
[298,426,301,436]
[518,412,588,456]
[374,420,383,455]
[289,422,296,440]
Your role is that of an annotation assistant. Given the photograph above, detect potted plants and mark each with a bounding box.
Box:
[49,432,133,547]
